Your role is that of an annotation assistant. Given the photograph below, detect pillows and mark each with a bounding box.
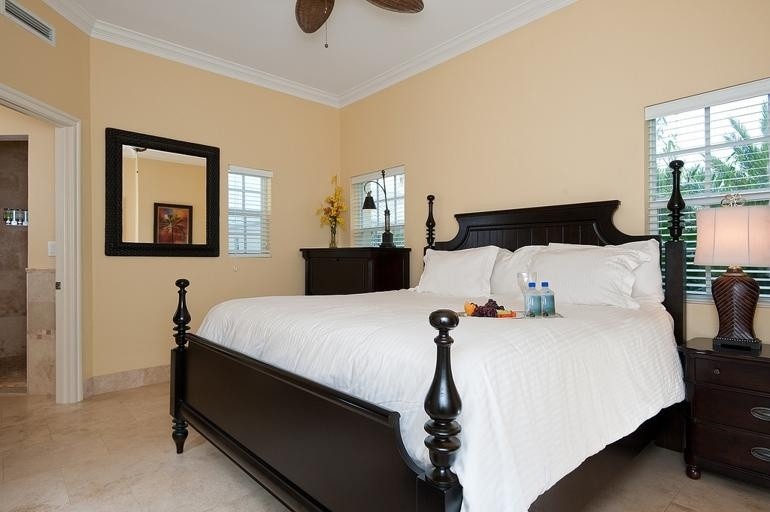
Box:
[549,238,664,303]
[490,248,514,298]
[415,245,499,297]
[511,245,646,309]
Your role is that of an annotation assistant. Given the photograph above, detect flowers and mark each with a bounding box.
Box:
[316,175,349,245]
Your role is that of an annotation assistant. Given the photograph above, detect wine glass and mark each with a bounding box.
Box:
[517,272,537,317]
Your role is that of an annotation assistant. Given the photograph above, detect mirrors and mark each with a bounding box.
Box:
[105,127,220,257]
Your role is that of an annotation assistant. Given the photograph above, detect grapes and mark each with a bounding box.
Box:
[470,298,505,318]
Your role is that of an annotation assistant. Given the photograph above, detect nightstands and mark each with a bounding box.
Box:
[677,336,769,490]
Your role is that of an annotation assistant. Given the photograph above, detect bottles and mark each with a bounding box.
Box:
[524,282,541,315]
[3,208,28,226]
[538,282,555,315]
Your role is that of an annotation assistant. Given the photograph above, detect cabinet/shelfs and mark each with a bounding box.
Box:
[299,246,412,296]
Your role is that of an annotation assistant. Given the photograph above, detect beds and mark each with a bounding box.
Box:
[168,160,686,512]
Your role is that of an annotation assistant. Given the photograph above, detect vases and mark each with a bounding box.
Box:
[330,216,336,249]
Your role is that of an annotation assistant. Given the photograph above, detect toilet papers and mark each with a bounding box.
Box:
[4,208,14,225]
[15,208,24,225]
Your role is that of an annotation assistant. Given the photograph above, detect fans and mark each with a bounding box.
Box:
[295,0,425,49]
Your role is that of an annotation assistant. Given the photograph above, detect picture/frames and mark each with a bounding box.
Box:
[154,203,193,245]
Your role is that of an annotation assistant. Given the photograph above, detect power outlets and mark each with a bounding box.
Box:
[47,240,56,257]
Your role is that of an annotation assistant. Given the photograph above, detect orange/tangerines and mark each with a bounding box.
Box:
[464,303,477,316]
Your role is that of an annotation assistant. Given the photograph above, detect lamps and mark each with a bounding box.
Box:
[362,170,396,248]
[4,207,28,228]
[693,193,770,351]
